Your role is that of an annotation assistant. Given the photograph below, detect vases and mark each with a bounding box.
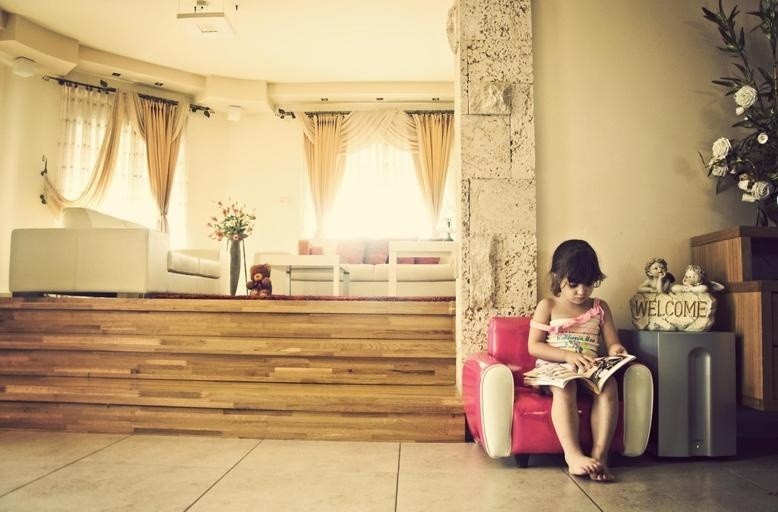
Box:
[225,233,249,295]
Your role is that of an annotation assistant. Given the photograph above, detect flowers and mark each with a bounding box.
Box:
[206,197,256,244]
[698,2,777,226]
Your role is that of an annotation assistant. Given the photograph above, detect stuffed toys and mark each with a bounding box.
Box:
[246,264,272,298]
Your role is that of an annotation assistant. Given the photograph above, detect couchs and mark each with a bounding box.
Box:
[9,206,221,300]
[256,245,456,298]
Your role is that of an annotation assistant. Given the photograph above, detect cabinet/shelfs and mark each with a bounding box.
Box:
[688,223,775,417]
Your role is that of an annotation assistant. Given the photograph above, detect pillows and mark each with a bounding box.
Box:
[297,238,442,264]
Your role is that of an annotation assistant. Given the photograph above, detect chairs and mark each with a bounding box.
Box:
[461,312,656,464]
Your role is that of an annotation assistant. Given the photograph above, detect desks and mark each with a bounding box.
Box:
[261,241,456,296]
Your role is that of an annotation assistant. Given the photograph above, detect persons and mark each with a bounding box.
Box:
[528,239,628,481]
[637,258,708,293]
